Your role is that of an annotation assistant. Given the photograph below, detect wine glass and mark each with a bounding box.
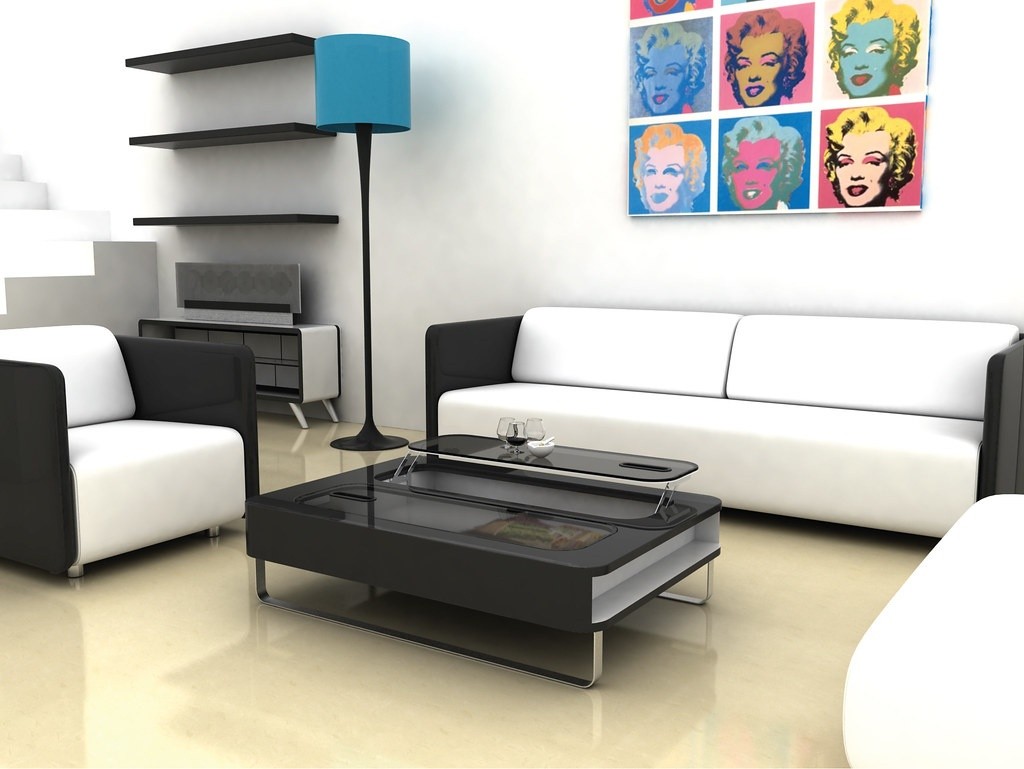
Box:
[506,421,528,454]
[497,417,518,450]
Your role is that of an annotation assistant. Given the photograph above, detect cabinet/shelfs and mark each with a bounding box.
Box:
[125,33,340,226]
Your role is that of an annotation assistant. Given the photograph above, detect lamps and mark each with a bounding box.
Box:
[313,33,411,452]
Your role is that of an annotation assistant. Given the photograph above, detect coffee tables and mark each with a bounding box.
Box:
[246,434,722,689]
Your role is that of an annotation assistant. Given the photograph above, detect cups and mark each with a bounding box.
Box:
[523,418,545,442]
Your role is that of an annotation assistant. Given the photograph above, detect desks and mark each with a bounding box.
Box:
[138,261,341,429]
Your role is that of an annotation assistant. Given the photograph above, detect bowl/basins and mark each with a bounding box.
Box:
[527,441,555,458]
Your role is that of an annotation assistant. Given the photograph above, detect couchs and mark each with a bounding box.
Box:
[424,306,1024,539]
[0,324,260,579]
[841,493,1024,768]
